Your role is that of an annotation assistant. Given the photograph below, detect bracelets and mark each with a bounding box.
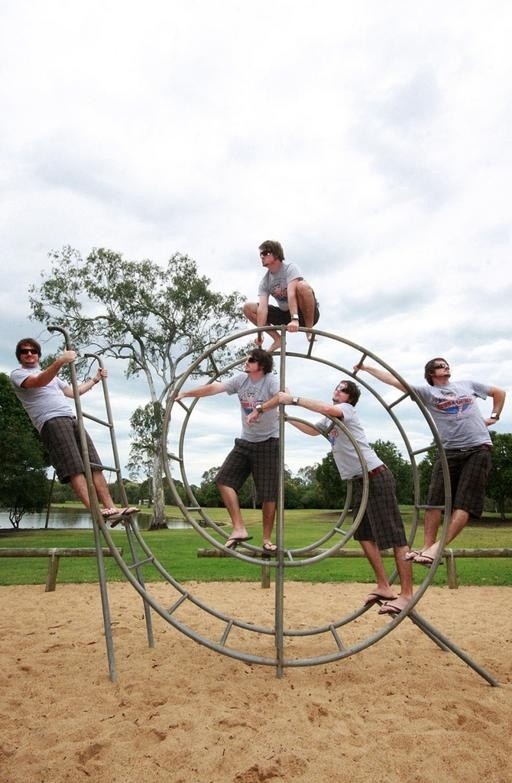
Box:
[92,377,99,385]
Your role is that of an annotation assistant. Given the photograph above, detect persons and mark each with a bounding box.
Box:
[352,356,507,564]
[243,239,320,352]
[10,337,142,529]
[168,348,292,551]
[278,380,414,614]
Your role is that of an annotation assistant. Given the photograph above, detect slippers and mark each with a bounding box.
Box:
[225,536,253,548]
[403,550,419,561]
[378,601,401,615]
[413,552,444,565]
[97,508,119,530]
[110,507,141,528]
[364,593,397,607]
[262,543,277,552]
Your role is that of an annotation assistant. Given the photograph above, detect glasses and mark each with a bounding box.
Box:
[248,357,257,363]
[433,363,446,369]
[260,250,273,257]
[335,385,348,394]
[21,348,39,354]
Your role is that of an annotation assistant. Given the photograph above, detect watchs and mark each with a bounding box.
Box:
[290,312,300,320]
[291,395,303,406]
[255,403,265,415]
[490,411,499,420]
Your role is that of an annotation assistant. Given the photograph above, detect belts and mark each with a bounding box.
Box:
[367,464,386,478]
[460,444,489,452]
